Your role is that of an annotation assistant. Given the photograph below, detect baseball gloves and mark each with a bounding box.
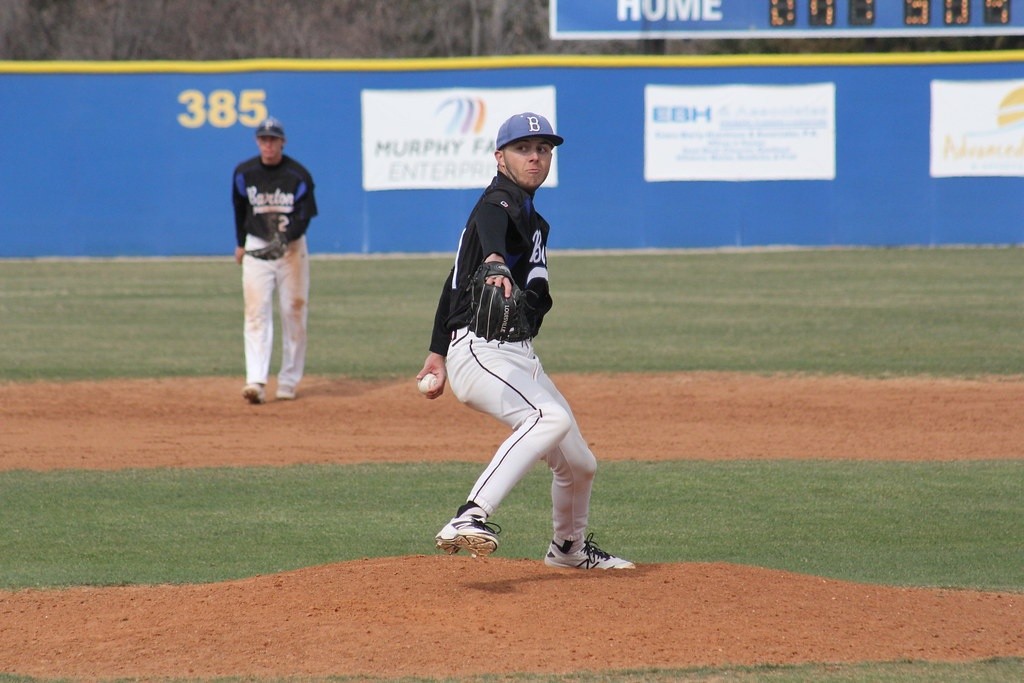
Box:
[467,261,532,343]
[244,231,288,260]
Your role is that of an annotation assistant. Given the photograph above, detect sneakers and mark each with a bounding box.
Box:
[434,507,501,558]
[545,532,636,569]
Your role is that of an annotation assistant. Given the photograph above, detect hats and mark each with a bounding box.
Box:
[496,112,564,150]
[255,119,284,139]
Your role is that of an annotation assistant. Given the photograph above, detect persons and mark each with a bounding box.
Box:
[232,117,317,404]
[417,113,636,570]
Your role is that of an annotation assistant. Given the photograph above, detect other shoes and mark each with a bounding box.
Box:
[276,391,295,400]
[242,383,264,404]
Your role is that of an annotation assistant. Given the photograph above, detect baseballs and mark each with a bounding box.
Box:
[417,373,438,394]
